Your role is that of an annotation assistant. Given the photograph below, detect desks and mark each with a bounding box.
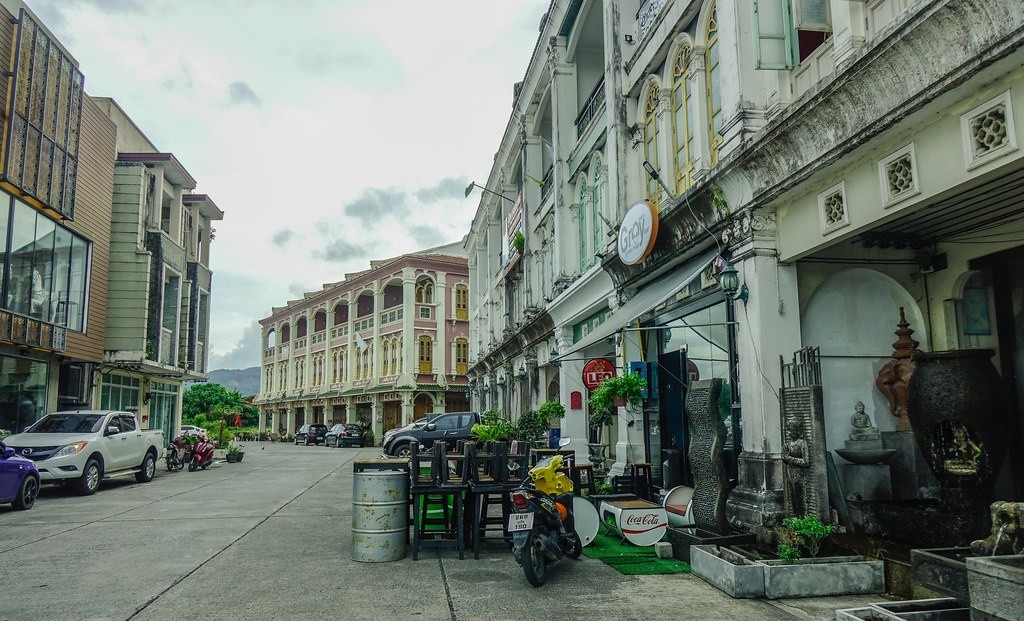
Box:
[10,275,25,312]
[58,300,79,329]
[531,448,577,496]
[354,458,515,561]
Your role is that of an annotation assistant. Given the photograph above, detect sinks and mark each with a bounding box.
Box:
[965,554,1024,621]
[867,597,969,615]
[910,547,983,603]
[835,607,906,621]
[895,608,1010,621]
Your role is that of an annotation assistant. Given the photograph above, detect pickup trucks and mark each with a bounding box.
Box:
[2,410,165,496]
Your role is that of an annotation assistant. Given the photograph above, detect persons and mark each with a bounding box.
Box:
[781,416,809,515]
[849,401,879,434]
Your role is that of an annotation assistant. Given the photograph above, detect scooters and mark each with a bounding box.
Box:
[188,428,217,471]
[507,436,583,586]
[165,430,188,472]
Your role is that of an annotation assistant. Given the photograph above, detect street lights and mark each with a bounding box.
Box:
[719,258,742,486]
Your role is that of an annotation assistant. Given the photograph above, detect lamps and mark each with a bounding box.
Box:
[606,335,621,347]
[625,35,632,44]
[663,324,672,350]
[143,393,151,404]
[719,258,749,306]
[474,388,479,397]
[466,391,471,401]
[519,365,527,379]
[550,347,562,368]
[499,375,506,388]
[643,161,675,200]
[465,181,515,204]
[484,382,490,393]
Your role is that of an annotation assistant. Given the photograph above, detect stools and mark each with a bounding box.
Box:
[575,464,595,497]
[631,463,654,499]
[588,493,638,521]
[410,438,531,541]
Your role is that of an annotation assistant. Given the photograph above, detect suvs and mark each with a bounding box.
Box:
[294,423,328,446]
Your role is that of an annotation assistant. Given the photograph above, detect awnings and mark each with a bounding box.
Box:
[550,244,739,363]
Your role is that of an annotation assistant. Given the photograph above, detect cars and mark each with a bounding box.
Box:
[324,424,367,447]
[0,440,41,510]
[383,412,510,459]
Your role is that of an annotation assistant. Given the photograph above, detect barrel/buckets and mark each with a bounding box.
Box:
[351,471,410,562]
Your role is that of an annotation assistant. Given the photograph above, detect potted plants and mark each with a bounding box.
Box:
[366,428,376,448]
[225,444,245,463]
[587,371,649,416]
[537,398,565,423]
[513,231,524,256]
[755,514,885,599]
